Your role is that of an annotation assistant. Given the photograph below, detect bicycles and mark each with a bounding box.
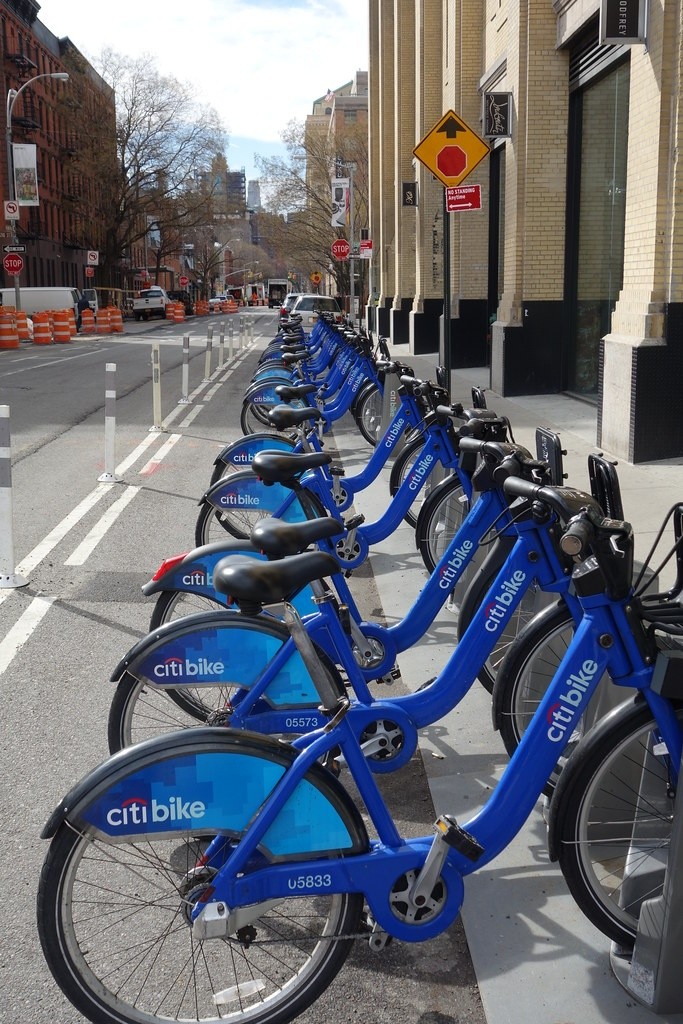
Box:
[34,305,683,1024]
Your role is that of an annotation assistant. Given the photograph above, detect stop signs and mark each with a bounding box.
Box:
[179,276,188,286]
[3,254,24,274]
[330,238,351,260]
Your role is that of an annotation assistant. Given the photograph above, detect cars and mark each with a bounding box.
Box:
[276,293,319,323]
[288,295,343,336]
[206,294,234,313]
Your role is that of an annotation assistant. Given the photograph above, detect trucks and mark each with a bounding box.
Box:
[267,278,292,309]
[0,287,99,320]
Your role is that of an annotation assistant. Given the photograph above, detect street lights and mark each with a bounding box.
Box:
[293,153,359,323]
[143,194,179,285]
[242,260,259,306]
[5,69,68,250]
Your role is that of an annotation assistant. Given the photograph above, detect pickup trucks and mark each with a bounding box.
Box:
[132,286,171,321]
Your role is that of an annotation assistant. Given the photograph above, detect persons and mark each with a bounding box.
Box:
[76,295,90,333]
